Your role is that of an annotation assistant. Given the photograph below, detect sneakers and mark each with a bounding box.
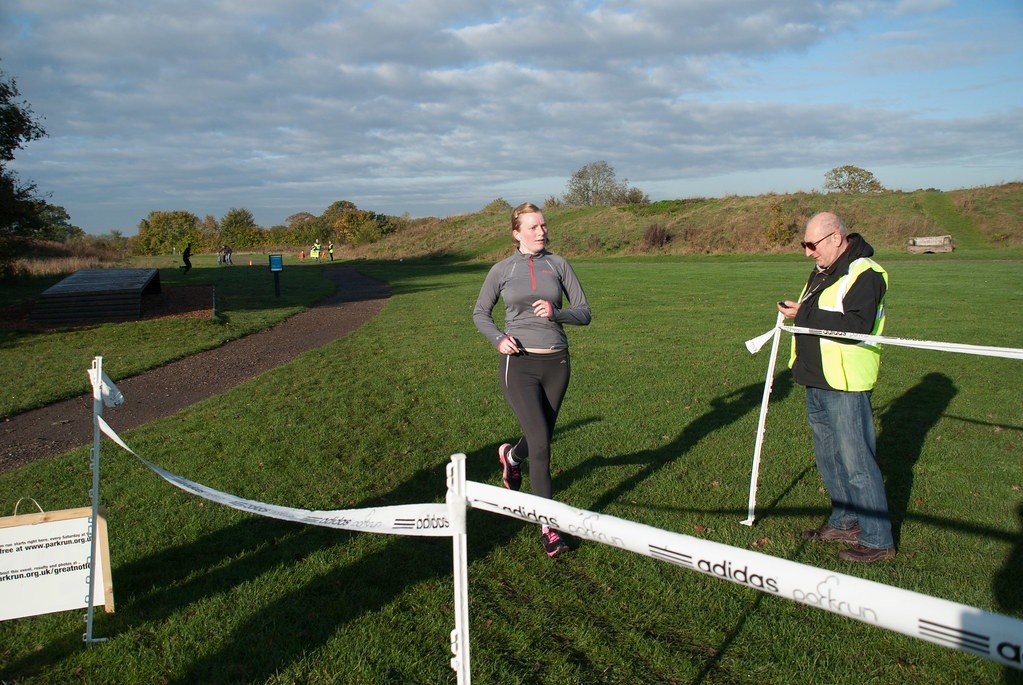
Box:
[498,443,523,491]
[838,542,896,563]
[801,520,862,544]
[537,528,569,558]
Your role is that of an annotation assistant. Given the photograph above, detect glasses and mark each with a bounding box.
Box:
[800,232,835,251]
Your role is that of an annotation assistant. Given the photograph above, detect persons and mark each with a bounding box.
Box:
[218,244,233,266]
[180,242,194,276]
[775,209,900,565]
[473,202,594,559]
[326,241,336,261]
[312,239,321,263]
[298,250,305,263]
